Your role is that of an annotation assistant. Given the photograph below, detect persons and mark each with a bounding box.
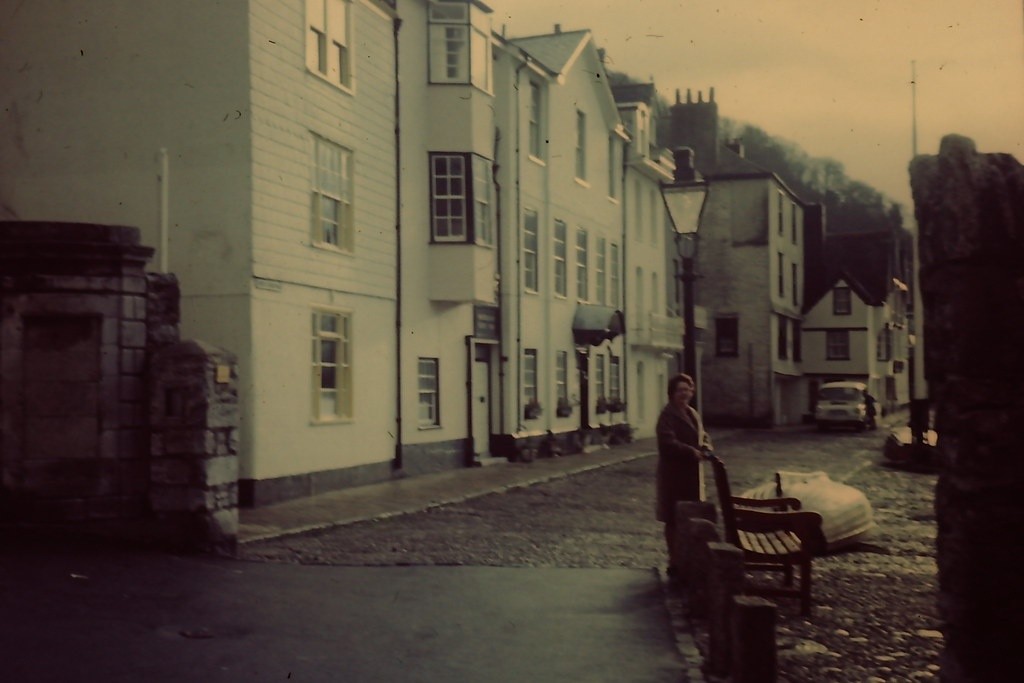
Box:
[862,389,878,431]
[907,400,929,444]
[656,374,714,579]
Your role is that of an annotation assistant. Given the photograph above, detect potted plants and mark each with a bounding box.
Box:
[524,398,543,419]
[596,395,624,415]
[556,397,573,417]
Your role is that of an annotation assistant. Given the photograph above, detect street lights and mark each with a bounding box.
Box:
[658,147,710,416]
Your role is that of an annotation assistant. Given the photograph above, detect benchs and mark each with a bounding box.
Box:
[710,453,831,611]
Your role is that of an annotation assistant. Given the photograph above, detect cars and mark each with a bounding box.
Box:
[815,381,868,433]
